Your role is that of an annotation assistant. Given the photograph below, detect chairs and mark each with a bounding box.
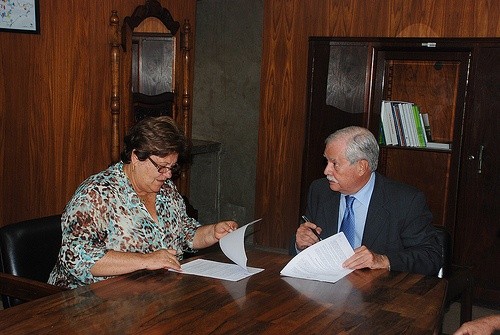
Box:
[0,214,69,309]
[432,223,473,326]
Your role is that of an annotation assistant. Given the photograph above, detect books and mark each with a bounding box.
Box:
[381,100,452,150]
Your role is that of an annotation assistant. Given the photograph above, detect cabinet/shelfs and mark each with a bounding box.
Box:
[110,0,220,221]
[298,36,500,311]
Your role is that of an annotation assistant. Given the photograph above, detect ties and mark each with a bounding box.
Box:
[339,196,355,250]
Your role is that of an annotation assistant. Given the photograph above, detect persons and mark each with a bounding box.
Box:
[289,126,446,277]
[46,114,238,290]
[452,314,500,335]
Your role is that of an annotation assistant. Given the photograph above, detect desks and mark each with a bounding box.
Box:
[0,245,449,335]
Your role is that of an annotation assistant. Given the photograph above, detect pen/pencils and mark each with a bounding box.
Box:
[302,215,322,240]
[175,252,185,256]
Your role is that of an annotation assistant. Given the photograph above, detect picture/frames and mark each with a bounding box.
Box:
[0,0,40,35]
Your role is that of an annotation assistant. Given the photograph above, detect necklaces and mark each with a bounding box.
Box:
[140,193,148,204]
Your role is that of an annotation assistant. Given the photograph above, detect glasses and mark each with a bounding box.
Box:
[148,156,181,173]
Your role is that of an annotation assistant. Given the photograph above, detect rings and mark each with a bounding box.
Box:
[170,259,172,262]
